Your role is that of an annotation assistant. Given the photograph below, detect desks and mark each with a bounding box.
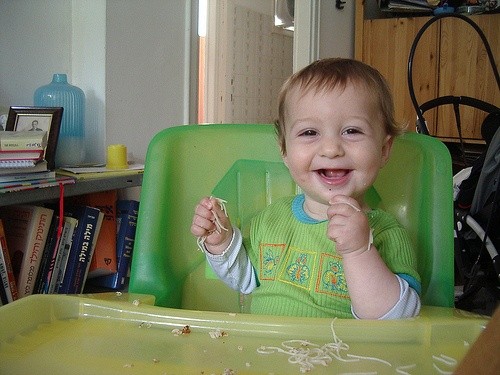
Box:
[0,293,492,375]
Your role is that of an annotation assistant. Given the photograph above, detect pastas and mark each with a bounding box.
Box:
[257,316,458,375]
[196,196,228,252]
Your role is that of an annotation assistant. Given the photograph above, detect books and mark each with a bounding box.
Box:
[0,131,140,307]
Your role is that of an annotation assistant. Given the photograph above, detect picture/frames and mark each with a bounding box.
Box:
[5,105,63,169]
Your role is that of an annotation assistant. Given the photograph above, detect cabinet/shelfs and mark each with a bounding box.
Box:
[353,0,500,144]
[1,148,145,305]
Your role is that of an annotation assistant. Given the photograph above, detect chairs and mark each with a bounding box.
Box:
[407,13,500,169]
[127,123,455,312]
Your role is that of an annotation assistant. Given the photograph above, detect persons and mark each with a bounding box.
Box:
[29,121,43,131]
[455,108,500,210]
[190,58,422,322]
